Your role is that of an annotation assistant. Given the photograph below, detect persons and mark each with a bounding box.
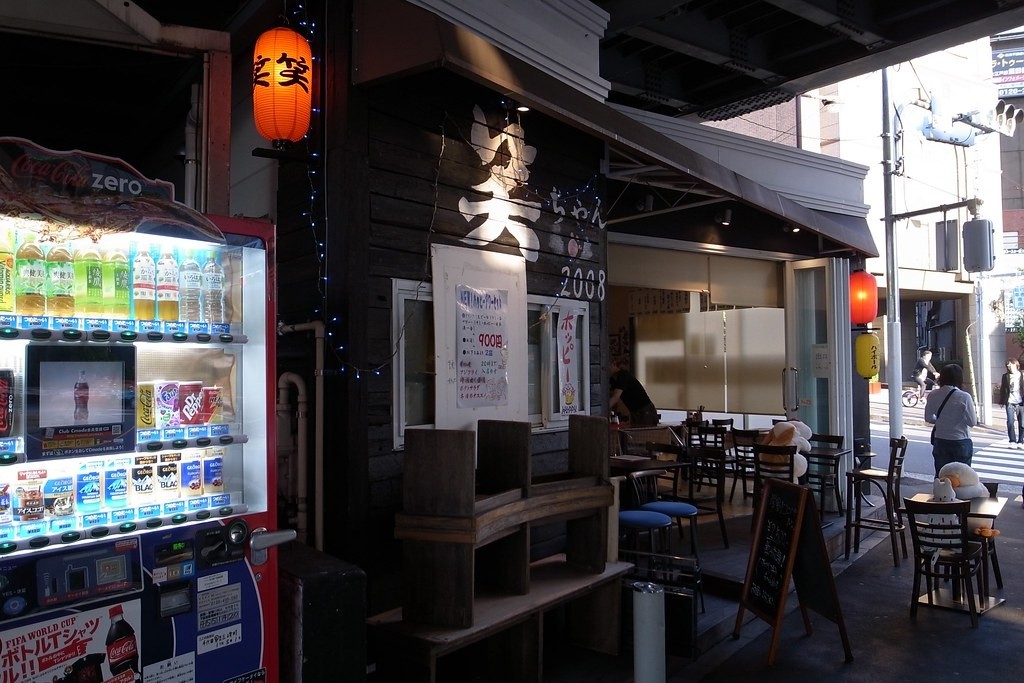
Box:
[607,356,661,430]
[1000,358,1024,449]
[924,363,975,476]
[911,348,940,396]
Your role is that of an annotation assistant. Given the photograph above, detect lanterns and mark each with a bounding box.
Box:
[850,268,878,328]
[250,29,314,149]
[856,332,880,379]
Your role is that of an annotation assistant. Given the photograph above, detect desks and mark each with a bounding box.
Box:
[609,454,692,512]
[910,492,1009,616]
[854,451,877,508]
[798,447,852,532]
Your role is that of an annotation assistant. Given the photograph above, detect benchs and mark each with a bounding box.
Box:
[390,412,612,629]
[646,442,730,548]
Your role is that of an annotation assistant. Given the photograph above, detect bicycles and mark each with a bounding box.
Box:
[902,376,941,408]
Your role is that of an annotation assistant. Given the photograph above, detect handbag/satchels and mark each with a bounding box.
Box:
[931,425,936,445]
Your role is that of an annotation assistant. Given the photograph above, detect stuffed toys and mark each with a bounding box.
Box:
[761,419,812,486]
[924,478,963,564]
[938,461,1002,539]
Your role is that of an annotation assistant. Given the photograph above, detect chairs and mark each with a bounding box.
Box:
[628,469,698,556]
[608,476,673,580]
[904,497,986,628]
[805,434,844,520]
[966,483,1005,590]
[844,436,908,570]
[681,420,709,478]
[750,443,797,538]
[696,426,738,495]
[729,427,761,505]
[617,429,657,495]
[712,419,734,474]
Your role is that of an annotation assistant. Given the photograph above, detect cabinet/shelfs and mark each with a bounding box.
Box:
[363,554,633,683]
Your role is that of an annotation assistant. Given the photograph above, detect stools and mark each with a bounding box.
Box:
[629,467,707,614]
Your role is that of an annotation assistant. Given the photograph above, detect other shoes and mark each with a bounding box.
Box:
[1008,442,1017,449]
[921,397,926,403]
[1016,443,1024,449]
